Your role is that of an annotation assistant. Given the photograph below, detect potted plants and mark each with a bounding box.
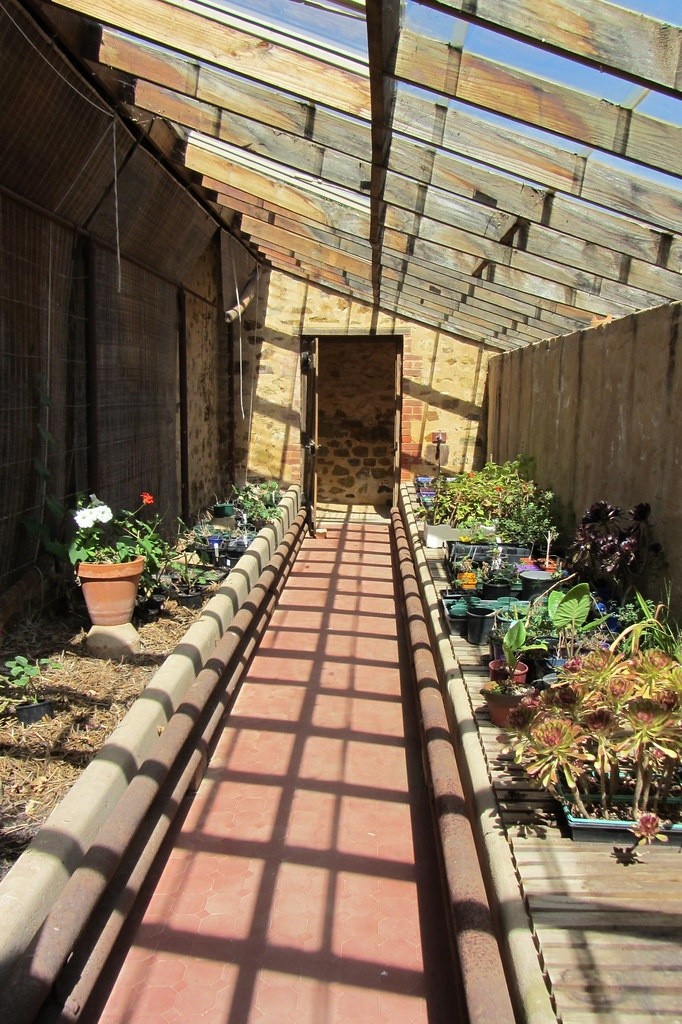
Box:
[160,472,284,612]
[413,438,682,846]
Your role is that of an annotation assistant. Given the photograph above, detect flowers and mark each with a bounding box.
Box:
[69,490,160,569]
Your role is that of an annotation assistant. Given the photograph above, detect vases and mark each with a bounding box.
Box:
[74,554,144,626]
